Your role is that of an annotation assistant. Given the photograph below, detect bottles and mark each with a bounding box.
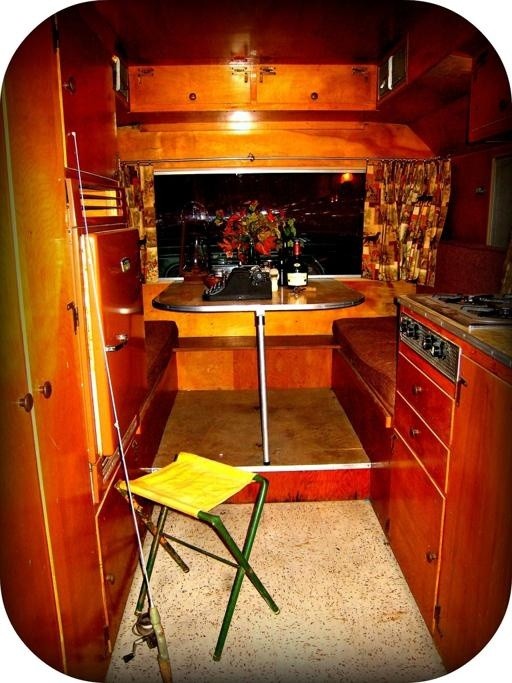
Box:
[285,239,309,289]
[278,234,291,286]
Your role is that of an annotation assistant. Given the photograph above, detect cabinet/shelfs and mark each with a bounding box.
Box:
[389,342,511,671]
[94,424,155,652]
[0,14,111,682]
[59,12,122,189]
[439,149,494,248]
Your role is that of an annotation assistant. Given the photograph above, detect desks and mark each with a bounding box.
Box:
[152,280,366,466]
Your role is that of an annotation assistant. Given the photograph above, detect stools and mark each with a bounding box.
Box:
[116,451,281,661]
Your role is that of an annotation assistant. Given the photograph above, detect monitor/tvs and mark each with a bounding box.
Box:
[154,169,366,281]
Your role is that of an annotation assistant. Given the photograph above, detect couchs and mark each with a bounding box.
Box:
[333,314,400,536]
[145,321,178,473]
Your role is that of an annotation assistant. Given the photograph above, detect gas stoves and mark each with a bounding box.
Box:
[406,291,512,331]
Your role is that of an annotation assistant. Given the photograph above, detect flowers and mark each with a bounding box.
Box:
[214,199,287,262]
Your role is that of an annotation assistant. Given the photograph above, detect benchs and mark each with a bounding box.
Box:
[172,336,341,351]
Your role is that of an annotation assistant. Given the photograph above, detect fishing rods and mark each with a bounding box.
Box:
[68,125,172,683]
